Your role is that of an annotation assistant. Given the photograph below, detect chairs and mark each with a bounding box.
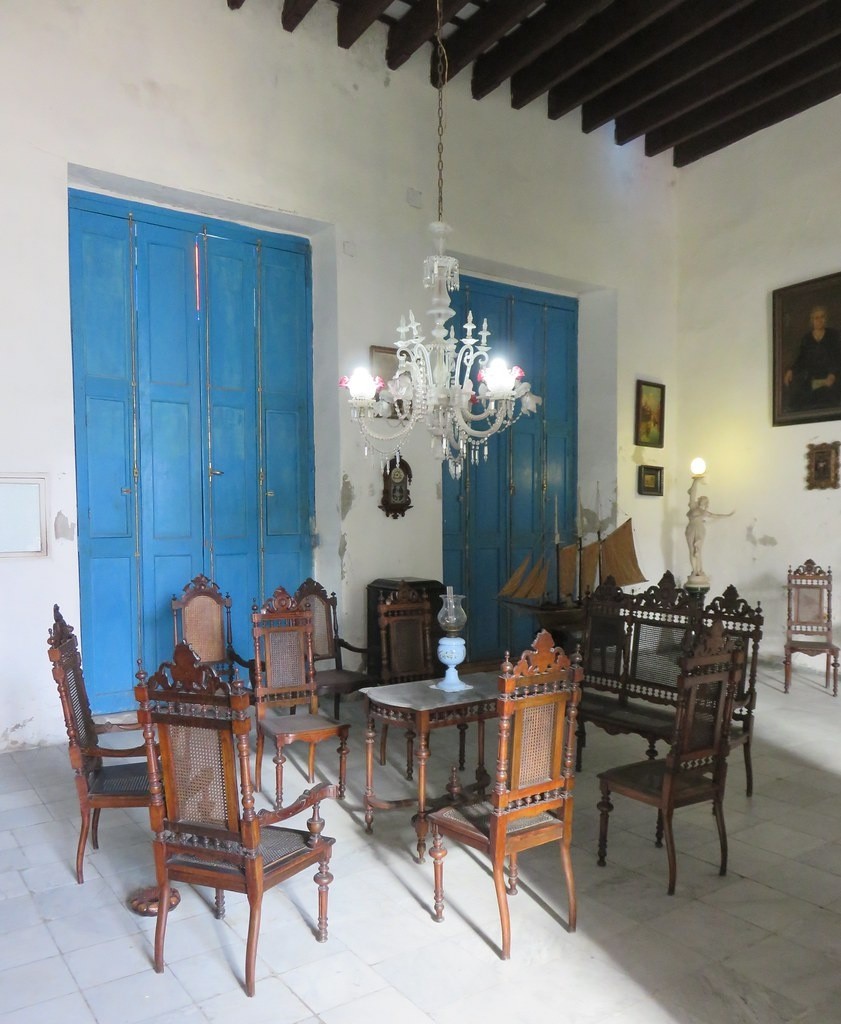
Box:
[373,578,469,780]
[132,638,337,999]
[252,585,351,813]
[596,620,746,896]
[48,603,192,884]
[784,558,841,697]
[426,630,584,961]
[290,577,381,719]
[171,573,267,707]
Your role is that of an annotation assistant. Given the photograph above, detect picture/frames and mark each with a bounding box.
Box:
[633,379,667,448]
[771,272,841,429]
[369,344,413,419]
[806,440,841,491]
[637,465,665,496]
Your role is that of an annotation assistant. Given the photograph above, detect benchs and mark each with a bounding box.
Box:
[574,572,765,818]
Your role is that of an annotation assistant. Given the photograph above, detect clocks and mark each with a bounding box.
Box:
[380,448,414,518]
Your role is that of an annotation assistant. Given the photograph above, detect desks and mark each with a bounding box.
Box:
[357,670,545,834]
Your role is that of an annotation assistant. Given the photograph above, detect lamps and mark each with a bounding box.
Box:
[676,457,735,668]
[338,1,542,486]
[436,586,468,693]
